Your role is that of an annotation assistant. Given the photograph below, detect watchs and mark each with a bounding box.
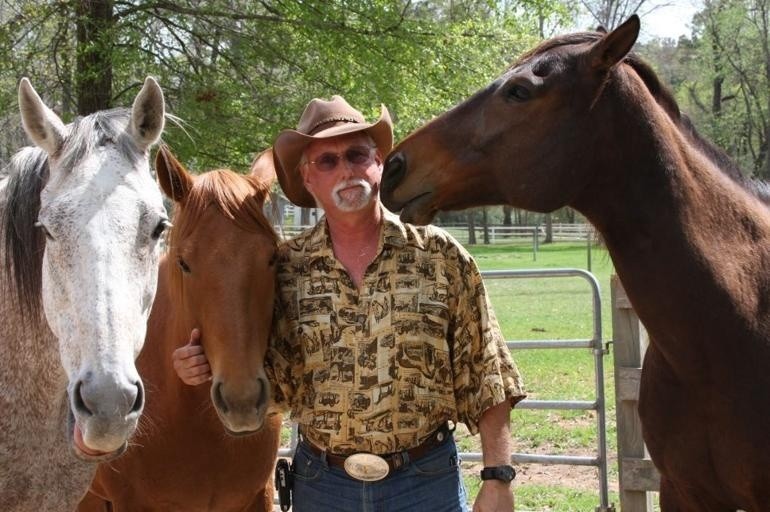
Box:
[479,463,517,483]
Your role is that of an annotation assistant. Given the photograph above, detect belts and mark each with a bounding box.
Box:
[295,420,455,485]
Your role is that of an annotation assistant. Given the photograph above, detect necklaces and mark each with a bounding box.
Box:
[331,226,379,258]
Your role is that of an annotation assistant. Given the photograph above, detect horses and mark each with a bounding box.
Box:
[0,76,194,511]
[378,12,770,511]
[75,141,282,512]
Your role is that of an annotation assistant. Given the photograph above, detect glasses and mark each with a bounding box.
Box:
[300,142,381,173]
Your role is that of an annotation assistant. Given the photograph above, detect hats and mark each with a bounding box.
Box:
[270,94,401,210]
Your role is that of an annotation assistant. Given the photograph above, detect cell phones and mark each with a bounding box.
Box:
[277,462,289,511]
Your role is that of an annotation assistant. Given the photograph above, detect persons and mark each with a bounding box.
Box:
[170,94,527,511]
